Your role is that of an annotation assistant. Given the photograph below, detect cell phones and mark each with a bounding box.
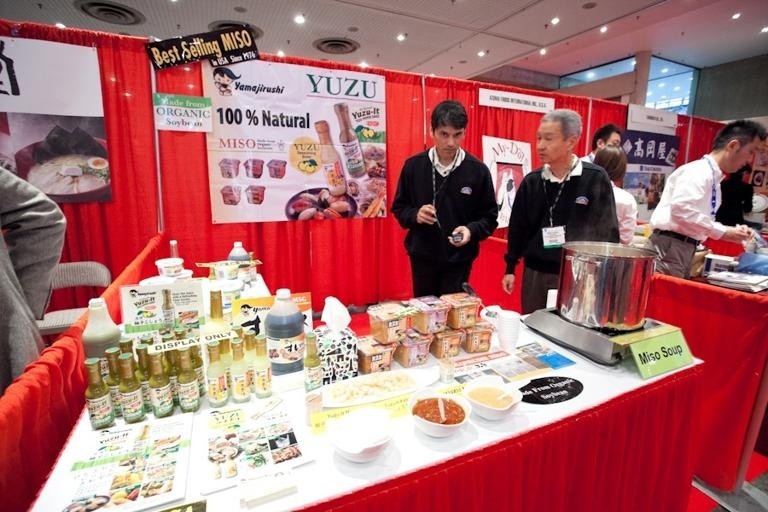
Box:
[453,232,463,243]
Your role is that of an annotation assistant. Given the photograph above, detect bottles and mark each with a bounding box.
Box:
[133,425,149,454]
[227,240,251,287]
[333,103,366,178]
[224,456,237,477]
[83,289,322,429]
[315,120,347,196]
[215,461,223,479]
[169,239,179,258]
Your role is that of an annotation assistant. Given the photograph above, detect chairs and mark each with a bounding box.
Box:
[36,260,111,347]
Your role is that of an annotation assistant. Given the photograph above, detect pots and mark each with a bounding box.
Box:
[556,241,659,333]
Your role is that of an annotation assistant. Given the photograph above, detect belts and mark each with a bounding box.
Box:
[653,228,701,246]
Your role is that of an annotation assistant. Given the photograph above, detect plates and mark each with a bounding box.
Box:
[286,188,357,219]
[751,192,768,212]
[753,171,764,186]
[62,496,110,512]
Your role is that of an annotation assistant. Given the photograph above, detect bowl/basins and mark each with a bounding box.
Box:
[406,387,472,438]
[325,409,392,463]
[463,375,523,421]
[701,237,744,256]
[15,138,110,206]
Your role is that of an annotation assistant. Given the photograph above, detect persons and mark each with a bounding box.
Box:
[501,110,621,315]
[577,123,621,164]
[592,145,638,246]
[712,162,755,227]
[0,164,68,397]
[646,118,767,280]
[389,97,498,298]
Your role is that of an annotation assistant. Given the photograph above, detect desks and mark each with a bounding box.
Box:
[30,311,702,512]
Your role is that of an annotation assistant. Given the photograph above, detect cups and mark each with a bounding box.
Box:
[498,310,520,350]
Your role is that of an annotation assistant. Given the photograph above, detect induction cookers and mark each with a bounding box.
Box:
[522,307,681,366]
